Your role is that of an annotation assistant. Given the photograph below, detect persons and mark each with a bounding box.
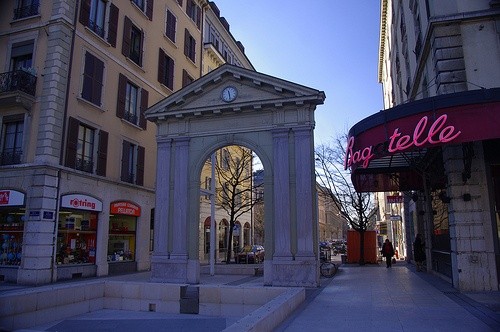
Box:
[414,234,425,272]
[382,239,394,268]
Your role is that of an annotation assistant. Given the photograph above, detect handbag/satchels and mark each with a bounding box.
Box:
[392,256,396,264]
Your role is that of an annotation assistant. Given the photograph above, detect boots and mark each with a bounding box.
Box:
[416,264,423,272]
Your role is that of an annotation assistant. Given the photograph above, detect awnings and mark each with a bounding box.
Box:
[345,86,500,192]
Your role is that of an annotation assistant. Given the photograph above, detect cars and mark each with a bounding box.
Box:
[234,245,265,264]
[319,238,347,261]
